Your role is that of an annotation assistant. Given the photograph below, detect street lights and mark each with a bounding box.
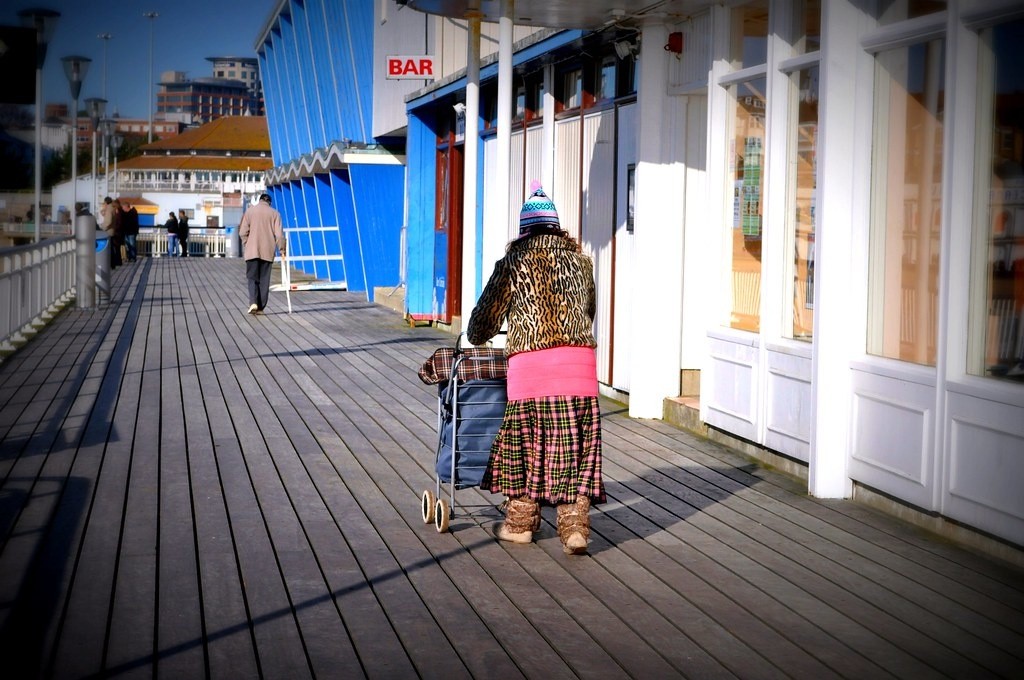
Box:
[84,96,108,211]
[110,133,128,199]
[99,32,112,122]
[99,118,116,201]
[62,53,93,237]
[141,12,162,143]
[26,6,61,243]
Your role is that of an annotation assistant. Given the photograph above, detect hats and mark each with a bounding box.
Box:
[260,194,271,202]
[519,180,560,239]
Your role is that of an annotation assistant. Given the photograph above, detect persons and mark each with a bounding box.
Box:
[239,193,286,315]
[26,208,33,220]
[466,180,607,555]
[102,197,139,269]
[160,211,189,257]
[75,203,100,230]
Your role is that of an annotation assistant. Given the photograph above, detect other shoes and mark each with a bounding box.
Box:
[248,304,263,315]
[563,531,587,555]
[492,521,532,544]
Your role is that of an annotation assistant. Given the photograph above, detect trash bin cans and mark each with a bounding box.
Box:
[95,230,111,305]
[225,224,239,258]
[57,206,70,224]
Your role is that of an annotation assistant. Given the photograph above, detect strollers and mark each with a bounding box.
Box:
[421,331,544,533]
